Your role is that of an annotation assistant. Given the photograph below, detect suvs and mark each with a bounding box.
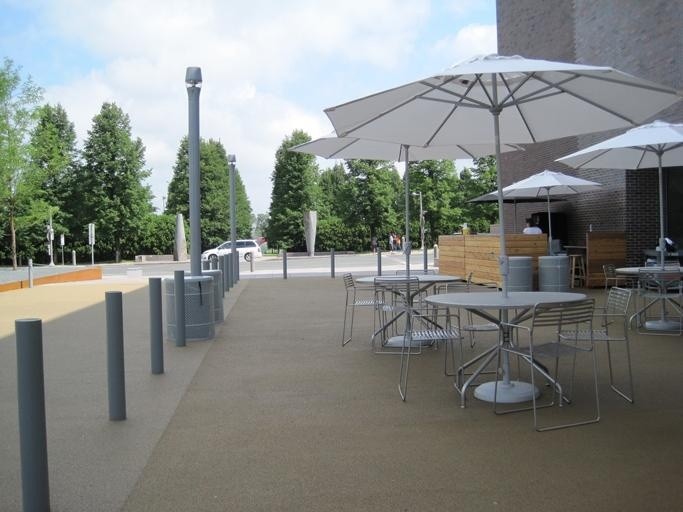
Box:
[202,239,262,262]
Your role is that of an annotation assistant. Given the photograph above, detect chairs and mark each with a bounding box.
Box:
[343,270,478,351]
[600,252,683,333]
[493,297,602,431]
[398,283,464,404]
[556,287,637,404]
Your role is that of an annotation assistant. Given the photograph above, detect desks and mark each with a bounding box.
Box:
[422,289,585,404]
[564,245,588,252]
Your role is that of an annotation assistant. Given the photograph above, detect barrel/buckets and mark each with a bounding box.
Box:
[537,255,571,292]
[506,257,533,291]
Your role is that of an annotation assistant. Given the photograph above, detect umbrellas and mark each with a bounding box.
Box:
[490,168,604,255]
[322,50,682,388]
[462,180,568,234]
[286,129,526,336]
[551,118,682,321]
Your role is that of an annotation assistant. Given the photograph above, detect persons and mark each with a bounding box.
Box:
[371,230,403,254]
[522,218,543,235]
